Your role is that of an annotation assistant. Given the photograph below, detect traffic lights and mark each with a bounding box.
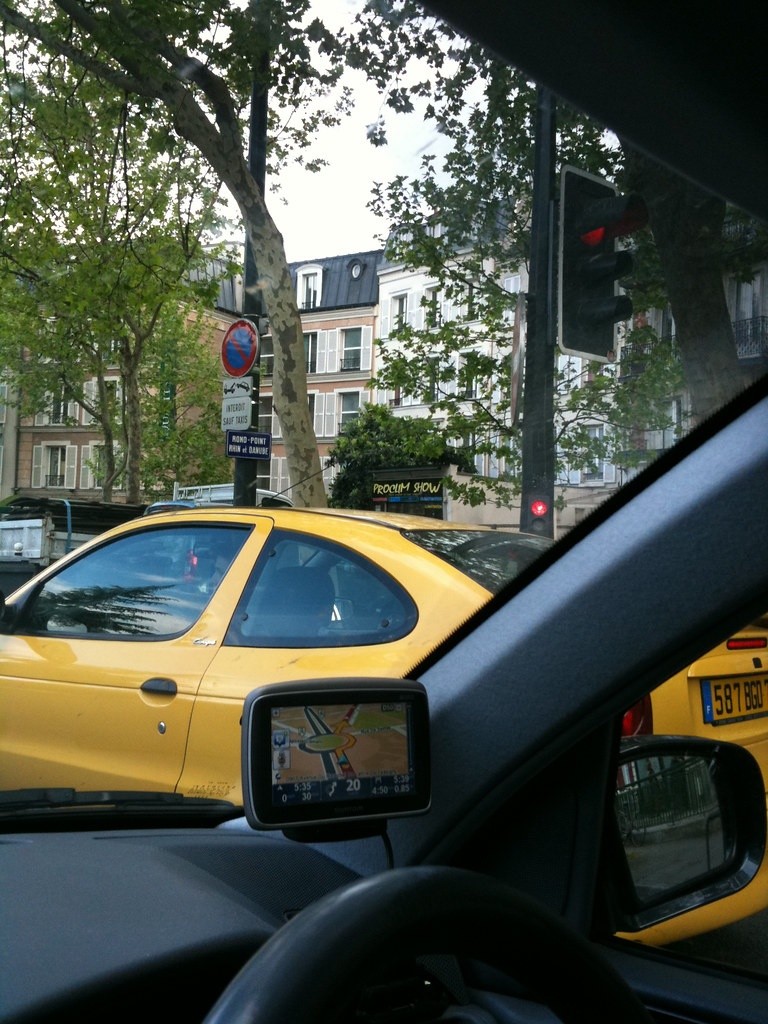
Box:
[560,164,636,361]
[525,489,552,540]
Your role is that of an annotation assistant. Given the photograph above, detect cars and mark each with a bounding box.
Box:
[0,495,767,954]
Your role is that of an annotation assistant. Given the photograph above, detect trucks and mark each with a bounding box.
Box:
[1,482,300,601]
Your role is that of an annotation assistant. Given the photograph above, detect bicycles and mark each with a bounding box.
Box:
[617,800,647,847]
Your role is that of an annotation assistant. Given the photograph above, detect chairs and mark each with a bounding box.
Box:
[249,564,335,638]
